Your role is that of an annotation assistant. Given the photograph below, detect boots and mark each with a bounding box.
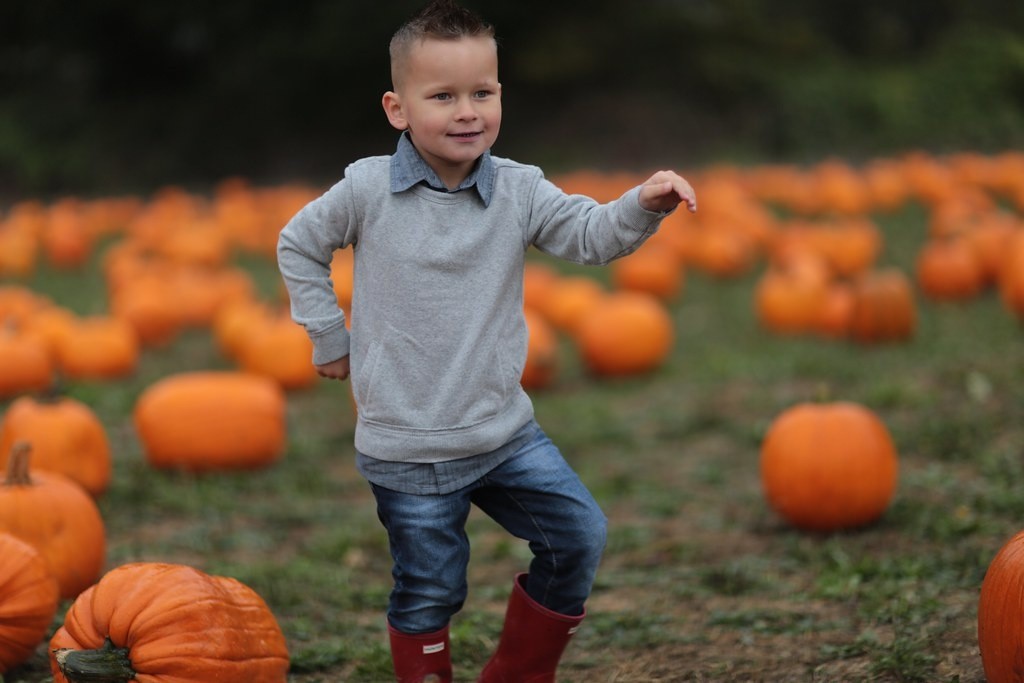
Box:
[474,572,586,680]
[387,618,453,682]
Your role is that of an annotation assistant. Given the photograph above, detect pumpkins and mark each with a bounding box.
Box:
[0,151,1024,682]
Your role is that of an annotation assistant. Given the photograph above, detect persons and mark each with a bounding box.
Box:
[276,4,701,682]
